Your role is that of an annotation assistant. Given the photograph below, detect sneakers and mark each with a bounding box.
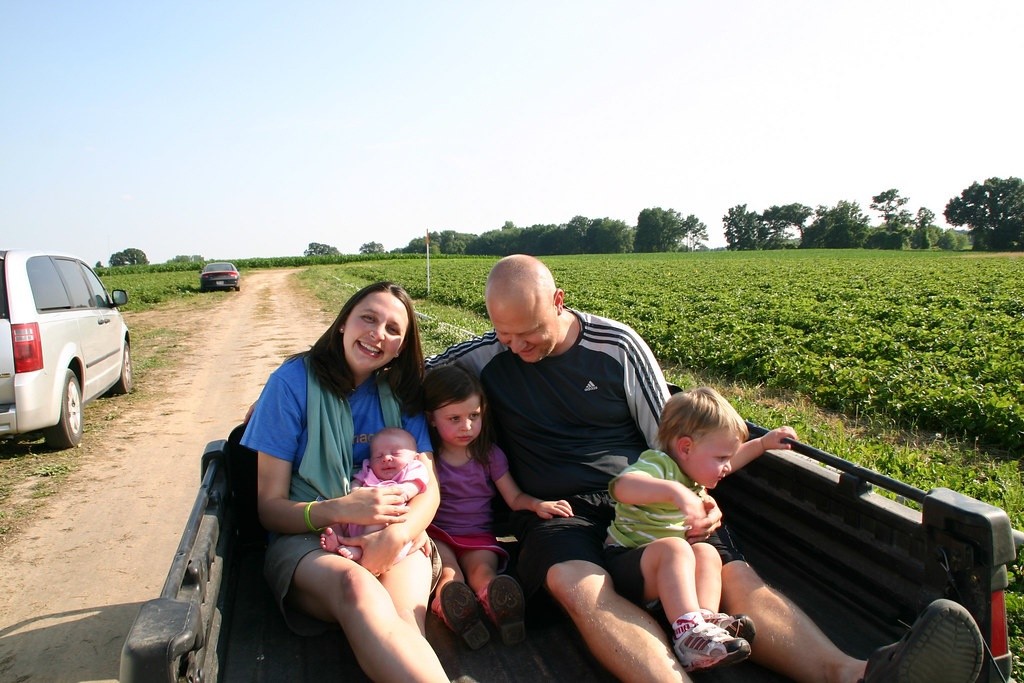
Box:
[673,622,751,672]
[702,613,756,644]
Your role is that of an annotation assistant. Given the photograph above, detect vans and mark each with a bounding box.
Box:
[0,250,133,449]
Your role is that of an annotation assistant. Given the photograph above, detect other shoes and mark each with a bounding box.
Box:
[431,580,491,650]
[857,599,984,683]
[477,575,526,646]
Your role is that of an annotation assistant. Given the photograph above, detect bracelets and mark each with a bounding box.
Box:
[305,501,323,533]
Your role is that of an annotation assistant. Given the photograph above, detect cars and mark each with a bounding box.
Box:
[200,261,241,293]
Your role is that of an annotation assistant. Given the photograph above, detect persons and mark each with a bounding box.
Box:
[239,284,452,683]
[428,254,985,683]
[320,427,429,566]
[406,365,574,651]
[600,386,799,674]
